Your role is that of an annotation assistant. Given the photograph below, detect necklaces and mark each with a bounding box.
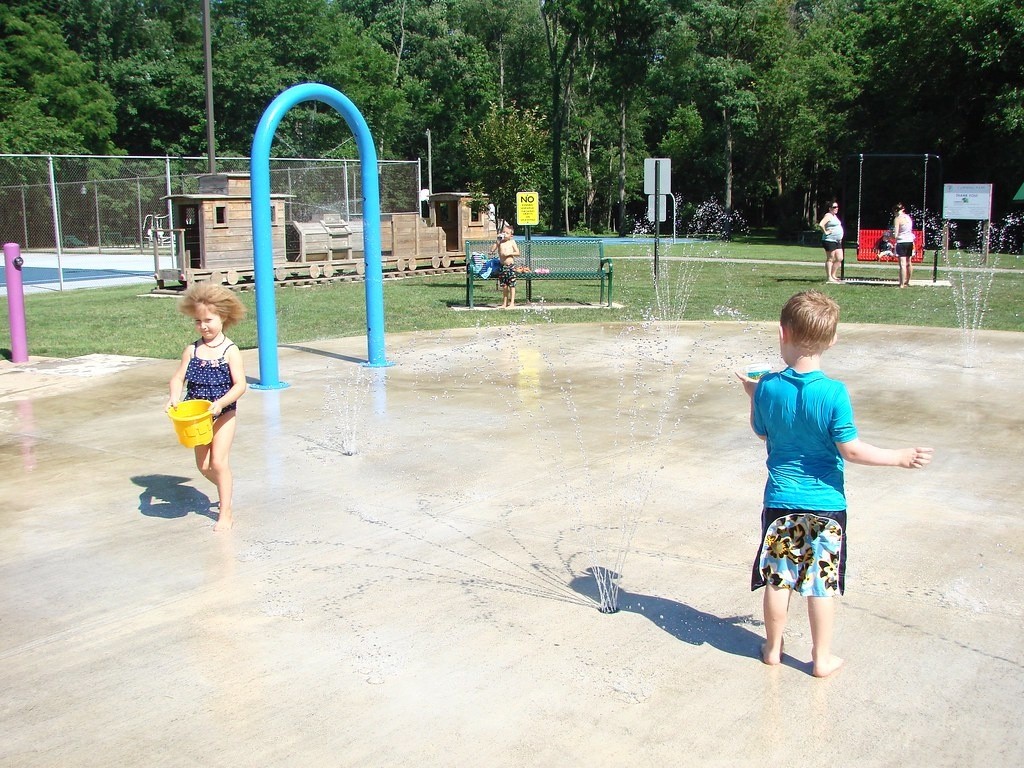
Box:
[202,335,226,348]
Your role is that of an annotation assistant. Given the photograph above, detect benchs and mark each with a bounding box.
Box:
[62,235,87,247]
[101,232,136,249]
[466,239,614,312]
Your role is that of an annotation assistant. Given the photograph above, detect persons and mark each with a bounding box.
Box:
[819,199,844,282]
[876,232,916,259]
[893,202,914,289]
[735,292,933,676]
[491,226,519,308]
[164,283,248,530]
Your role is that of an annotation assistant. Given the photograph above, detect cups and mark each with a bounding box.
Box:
[748,370,771,379]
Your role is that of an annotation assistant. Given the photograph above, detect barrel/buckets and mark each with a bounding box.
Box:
[167,399,214,447]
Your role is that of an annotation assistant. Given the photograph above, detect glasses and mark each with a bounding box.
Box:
[831,206,839,209]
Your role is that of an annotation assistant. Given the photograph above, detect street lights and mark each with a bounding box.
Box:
[425,127,433,195]
